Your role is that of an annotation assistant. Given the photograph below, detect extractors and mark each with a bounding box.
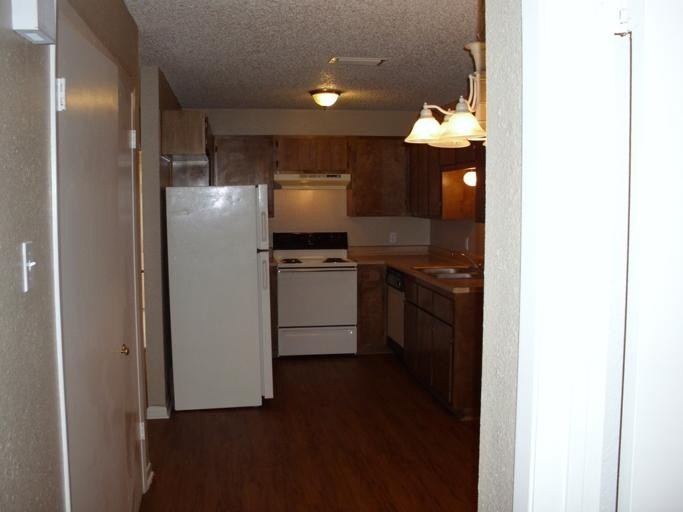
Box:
[273,171,351,191]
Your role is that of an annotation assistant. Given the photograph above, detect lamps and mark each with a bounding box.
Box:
[404,42,487,150]
[307,86,343,112]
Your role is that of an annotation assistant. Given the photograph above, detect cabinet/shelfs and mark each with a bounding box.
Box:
[437,97,478,172]
[401,276,483,421]
[167,135,214,186]
[213,134,274,219]
[161,109,210,161]
[344,136,410,217]
[358,263,388,354]
[272,134,348,175]
[404,109,474,220]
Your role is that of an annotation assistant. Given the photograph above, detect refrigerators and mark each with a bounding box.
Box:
[164,183,273,412]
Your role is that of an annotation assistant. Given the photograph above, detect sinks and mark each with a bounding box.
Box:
[417,267,482,279]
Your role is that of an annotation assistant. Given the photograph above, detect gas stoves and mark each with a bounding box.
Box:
[273,255,357,268]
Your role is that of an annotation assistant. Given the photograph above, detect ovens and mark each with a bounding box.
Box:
[275,263,358,358]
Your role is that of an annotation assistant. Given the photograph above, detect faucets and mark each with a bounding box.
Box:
[451,250,481,271]
